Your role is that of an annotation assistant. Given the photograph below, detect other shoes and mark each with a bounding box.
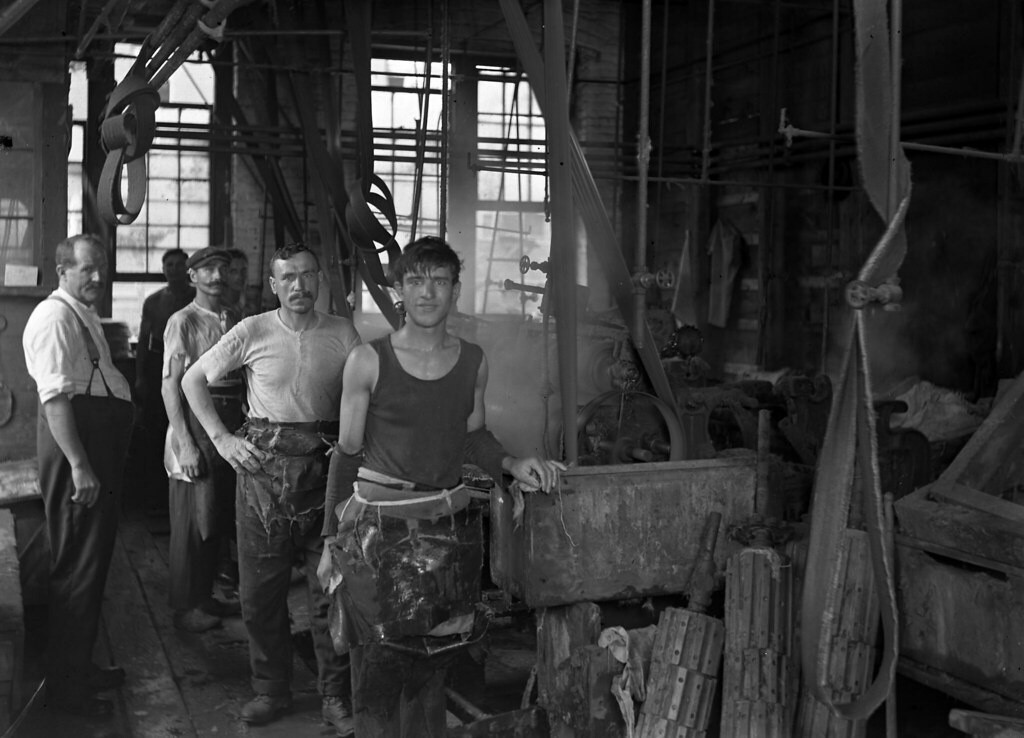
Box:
[173,607,221,634]
[202,596,242,617]
[54,693,113,717]
[76,666,125,689]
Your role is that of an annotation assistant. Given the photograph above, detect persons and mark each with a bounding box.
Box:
[181,242,363,738]
[135,248,196,444]
[160,247,249,635]
[316,236,568,738]
[21,232,134,718]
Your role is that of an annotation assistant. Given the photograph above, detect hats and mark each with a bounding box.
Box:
[186,246,231,269]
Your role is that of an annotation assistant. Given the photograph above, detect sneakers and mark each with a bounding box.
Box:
[321,695,355,738]
[241,694,292,725]
